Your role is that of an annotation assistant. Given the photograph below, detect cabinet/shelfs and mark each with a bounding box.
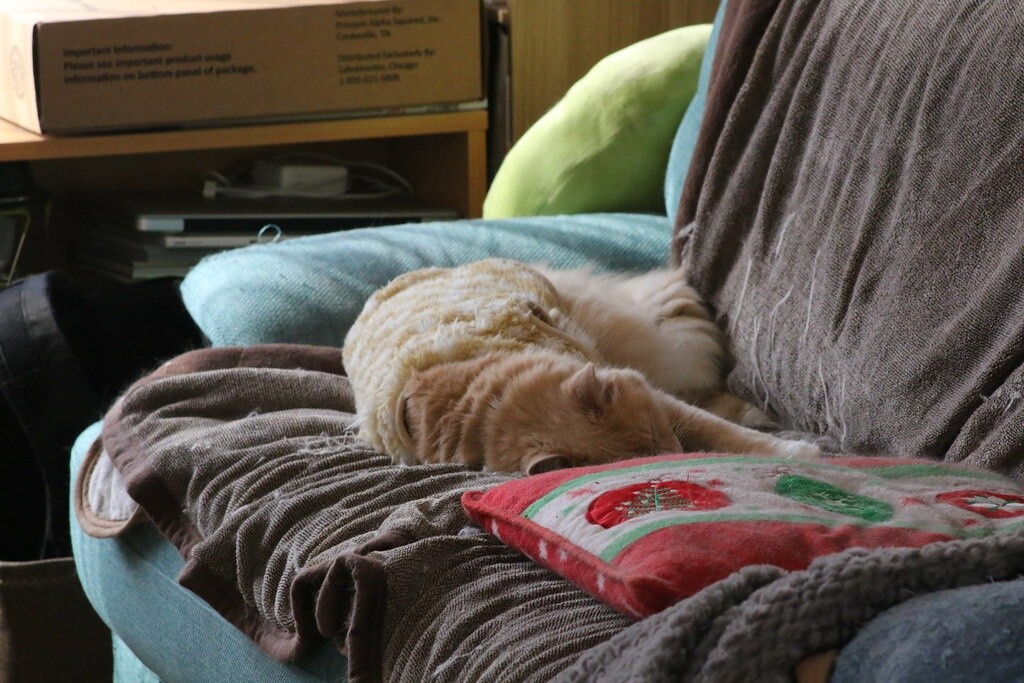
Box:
[0,111,489,219]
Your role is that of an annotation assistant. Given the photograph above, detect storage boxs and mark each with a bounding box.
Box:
[0,0,483,136]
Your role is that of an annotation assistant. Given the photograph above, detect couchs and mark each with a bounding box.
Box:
[68,0,1024,683]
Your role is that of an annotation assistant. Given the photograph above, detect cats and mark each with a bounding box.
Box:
[340,258,823,477]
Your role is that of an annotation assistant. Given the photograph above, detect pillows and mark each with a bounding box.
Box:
[460,455,1024,618]
